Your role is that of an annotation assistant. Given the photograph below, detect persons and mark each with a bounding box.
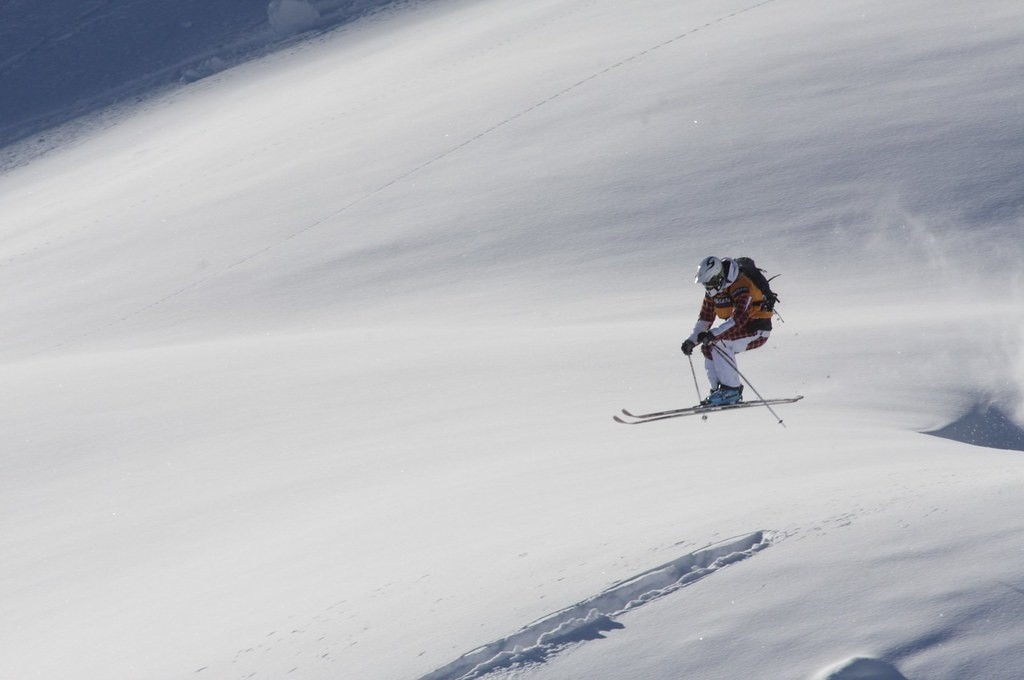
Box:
[681,256,774,410]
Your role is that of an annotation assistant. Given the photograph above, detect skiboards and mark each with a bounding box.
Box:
[613,396,804,424]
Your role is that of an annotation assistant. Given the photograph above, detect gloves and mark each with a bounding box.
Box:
[697,331,714,343]
[680,340,695,354]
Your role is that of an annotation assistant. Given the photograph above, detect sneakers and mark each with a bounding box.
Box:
[701,384,746,406]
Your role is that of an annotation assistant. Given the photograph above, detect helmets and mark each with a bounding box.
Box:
[695,258,728,296]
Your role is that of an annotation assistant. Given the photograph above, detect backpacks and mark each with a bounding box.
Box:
[733,256,778,311]
